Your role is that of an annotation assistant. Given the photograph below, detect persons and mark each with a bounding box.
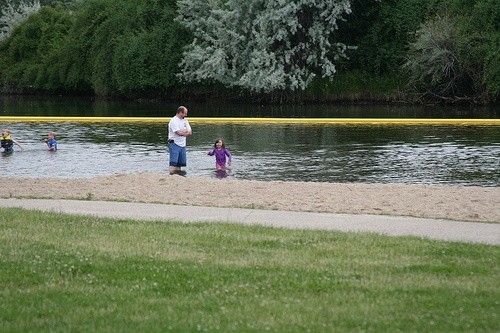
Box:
[207,138,232,170]
[44,132,58,152]
[0,127,25,153]
[168,105,192,170]
